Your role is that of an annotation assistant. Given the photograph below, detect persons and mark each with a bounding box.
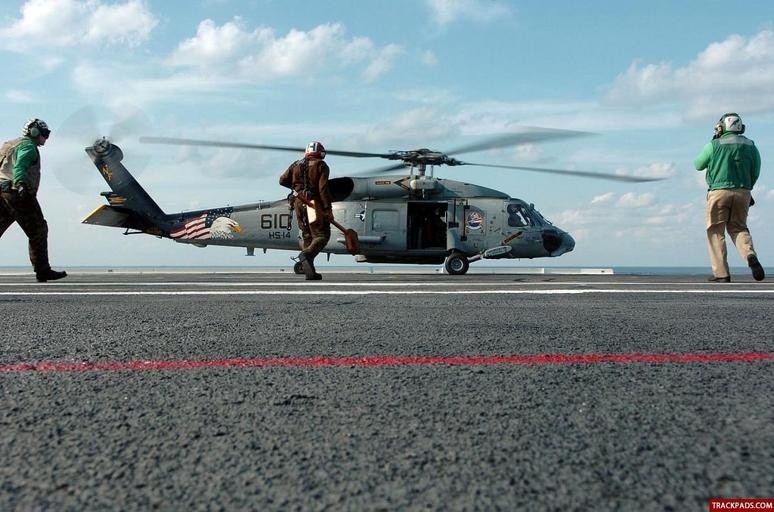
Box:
[693,112,764,283]
[278,140,334,280]
[0,117,69,282]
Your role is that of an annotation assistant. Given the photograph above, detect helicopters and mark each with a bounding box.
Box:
[81,129,664,275]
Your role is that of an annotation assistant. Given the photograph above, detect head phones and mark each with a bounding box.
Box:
[320,150,326,159]
[30,119,40,137]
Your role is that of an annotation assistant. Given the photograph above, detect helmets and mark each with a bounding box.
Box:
[21,117,51,144]
[304,141,326,159]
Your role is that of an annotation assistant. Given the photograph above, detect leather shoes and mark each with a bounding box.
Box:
[35,271,67,283]
[299,252,316,276]
[747,254,765,281]
[305,272,322,281]
[708,274,731,283]
